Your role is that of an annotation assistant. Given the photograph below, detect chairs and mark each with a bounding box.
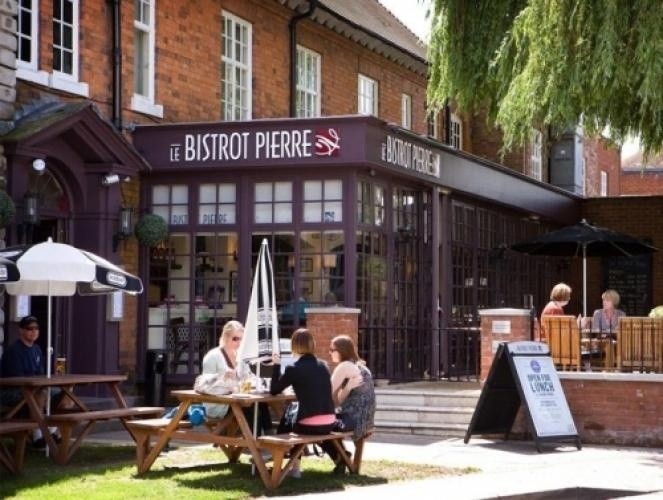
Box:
[541,315,663,372]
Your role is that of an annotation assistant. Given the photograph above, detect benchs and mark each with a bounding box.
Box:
[2,421,41,465]
[258,430,373,491]
[125,417,212,476]
[44,408,168,462]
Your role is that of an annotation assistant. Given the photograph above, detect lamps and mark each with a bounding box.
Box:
[112,207,136,252]
[17,191,38,245]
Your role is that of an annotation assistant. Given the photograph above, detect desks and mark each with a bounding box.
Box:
[165,390,299,490]
[1,374,128,466]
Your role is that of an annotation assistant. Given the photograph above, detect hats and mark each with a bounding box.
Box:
[18,316,38,327]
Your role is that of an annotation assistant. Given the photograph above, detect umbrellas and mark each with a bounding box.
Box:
[0,237,142,457]
[236,240,281,475]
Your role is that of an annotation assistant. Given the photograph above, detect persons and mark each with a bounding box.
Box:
[326,336,375,474]
[0,316,85,452]
[202,320,275,461]
[541,283,571,315]
[591,290,626,333]
[648,305,663,319]
[271,328,336,478]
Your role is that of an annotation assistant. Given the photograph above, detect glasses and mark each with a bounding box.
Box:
[230,336,241,341]
[26,326,39,331]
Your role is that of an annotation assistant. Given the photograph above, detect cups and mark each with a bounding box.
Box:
[54,358,66,375]
[240,381,251,395]
